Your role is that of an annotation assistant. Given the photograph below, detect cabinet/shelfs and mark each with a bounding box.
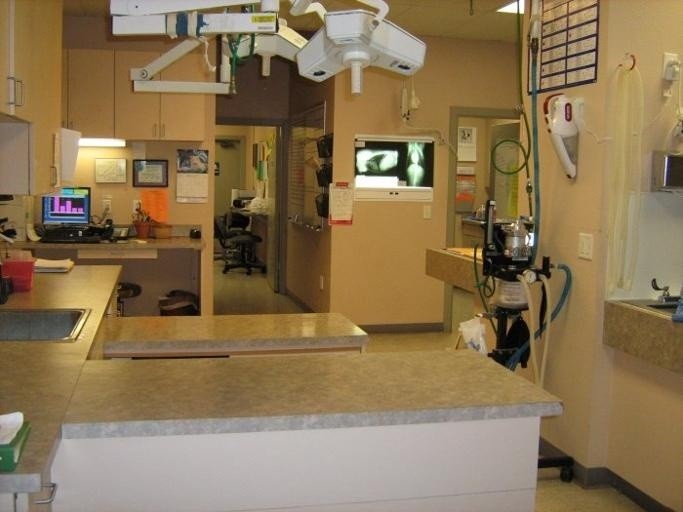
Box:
[0,0,61,196]
[62,48,113,140]
[114,50,204,140]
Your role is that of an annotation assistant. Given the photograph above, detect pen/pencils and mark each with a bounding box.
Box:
[137,209,150,223]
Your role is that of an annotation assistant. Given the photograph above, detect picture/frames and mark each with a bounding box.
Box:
[132,159,169,187]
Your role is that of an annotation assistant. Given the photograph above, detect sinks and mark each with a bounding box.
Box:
[648,303,678,316]
[0,306,91,343]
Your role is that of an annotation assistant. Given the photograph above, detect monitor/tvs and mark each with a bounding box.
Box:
[39,186,91,229]
[352,135,435,202]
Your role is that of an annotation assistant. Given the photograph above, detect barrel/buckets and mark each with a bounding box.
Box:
[3,258,36,291]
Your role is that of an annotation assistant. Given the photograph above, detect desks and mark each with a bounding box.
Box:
[0,223,209,317]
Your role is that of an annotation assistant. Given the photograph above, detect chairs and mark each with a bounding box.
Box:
[215,211,266,278]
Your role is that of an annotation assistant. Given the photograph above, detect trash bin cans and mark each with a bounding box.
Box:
[159,289,199,316]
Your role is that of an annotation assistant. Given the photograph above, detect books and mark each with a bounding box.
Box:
[0,420,31,471]
[33,256,74,273]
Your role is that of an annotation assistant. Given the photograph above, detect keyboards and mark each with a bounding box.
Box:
[42,232,101,244]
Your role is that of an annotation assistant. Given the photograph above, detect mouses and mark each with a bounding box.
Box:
[110,236,117,242]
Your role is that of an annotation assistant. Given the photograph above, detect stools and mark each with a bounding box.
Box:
[116,282,142,316]
